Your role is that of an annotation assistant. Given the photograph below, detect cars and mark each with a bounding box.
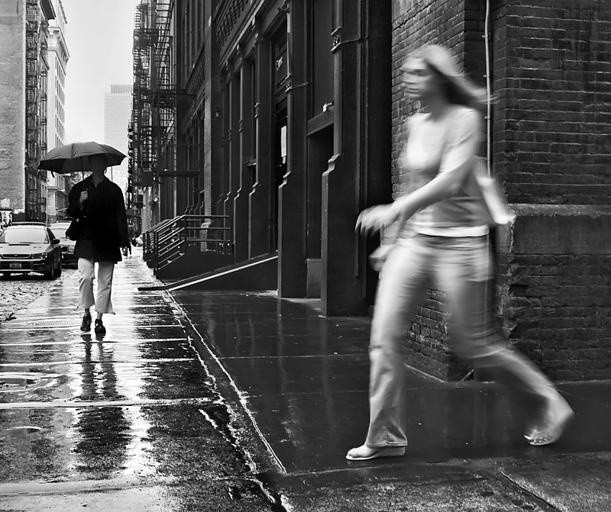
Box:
[0,221,77,278]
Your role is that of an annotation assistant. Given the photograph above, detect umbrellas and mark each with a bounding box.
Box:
[37,142,126,213]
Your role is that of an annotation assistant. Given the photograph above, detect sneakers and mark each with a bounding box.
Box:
[95,319,105,334]
[80,313,92,331]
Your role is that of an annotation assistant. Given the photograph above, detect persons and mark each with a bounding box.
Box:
[346,44,575,462]
[65,154,131,332]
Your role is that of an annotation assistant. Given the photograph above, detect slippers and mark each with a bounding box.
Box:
[344,444,406,460]
[527,411,571,447]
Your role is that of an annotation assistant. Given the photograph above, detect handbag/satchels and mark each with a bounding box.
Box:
[65,220,80,242]
